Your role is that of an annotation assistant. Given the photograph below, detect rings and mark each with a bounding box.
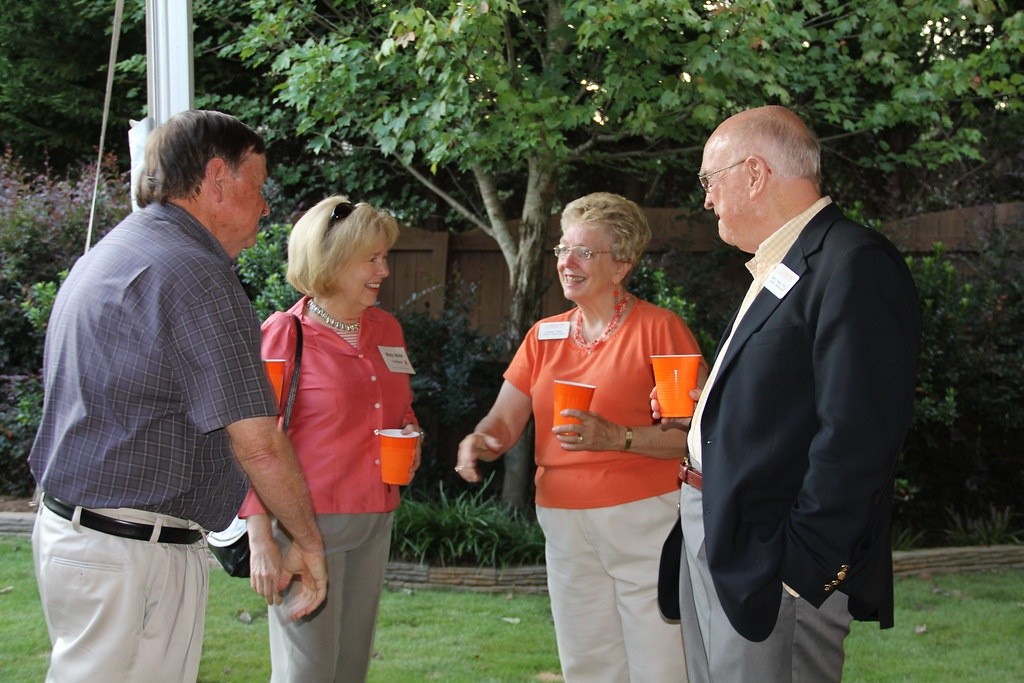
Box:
[577,433,583,444]
[455,465,465,472]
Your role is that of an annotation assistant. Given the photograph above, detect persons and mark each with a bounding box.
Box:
[26,110,328,683]
[238,193,422,683]
[649,106,922,683]
[456,192,709,683]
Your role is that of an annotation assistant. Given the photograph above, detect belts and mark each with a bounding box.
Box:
[677,453,702,490]
[42,492,209,544]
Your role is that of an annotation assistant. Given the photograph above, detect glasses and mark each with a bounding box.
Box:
[699,158,746,192]
[322,194,369,244]
[554,243,614,262]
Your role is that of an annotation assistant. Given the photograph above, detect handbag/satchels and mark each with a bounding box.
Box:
[206,512,249,577]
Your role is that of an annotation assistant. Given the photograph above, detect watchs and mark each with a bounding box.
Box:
[621,426,633,451]
[419,427,425,443]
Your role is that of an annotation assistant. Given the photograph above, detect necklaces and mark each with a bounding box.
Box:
[306,299,360,332]
[574,291,632,353]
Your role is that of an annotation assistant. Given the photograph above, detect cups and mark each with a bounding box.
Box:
[261,359,286,407]
[378,428,420,486]
[553,379,597,437]
[649,354,702,418]
